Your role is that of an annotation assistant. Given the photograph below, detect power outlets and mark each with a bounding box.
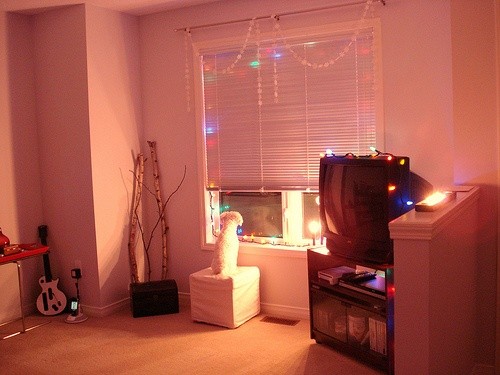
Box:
[74,261,82,276]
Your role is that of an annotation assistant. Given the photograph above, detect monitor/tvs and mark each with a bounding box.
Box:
[319,155,433,262]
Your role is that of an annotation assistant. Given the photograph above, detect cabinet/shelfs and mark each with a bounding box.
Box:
[307,246,395,375]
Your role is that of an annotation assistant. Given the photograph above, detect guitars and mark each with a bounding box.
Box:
[36,225,67,316]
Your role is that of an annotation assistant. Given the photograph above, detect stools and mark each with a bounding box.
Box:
[189,266,260,329]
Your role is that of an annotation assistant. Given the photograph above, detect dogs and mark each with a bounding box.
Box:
[211,211,242,282]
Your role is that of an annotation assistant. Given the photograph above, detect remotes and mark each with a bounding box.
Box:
[351,271,377,281]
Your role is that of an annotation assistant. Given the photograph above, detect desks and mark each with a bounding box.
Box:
[0,245,51,339]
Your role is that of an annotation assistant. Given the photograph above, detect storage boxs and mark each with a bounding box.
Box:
[130,280,179,318]
